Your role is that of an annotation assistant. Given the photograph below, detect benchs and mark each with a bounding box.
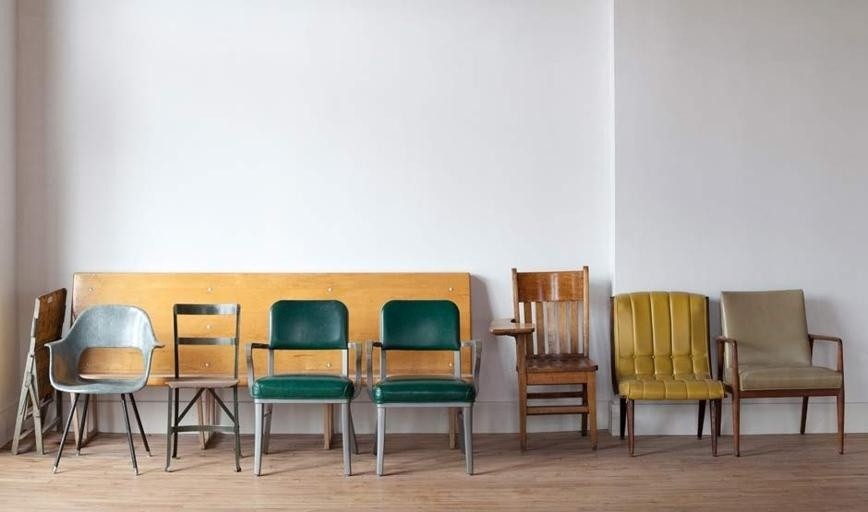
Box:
[69,272,477,454]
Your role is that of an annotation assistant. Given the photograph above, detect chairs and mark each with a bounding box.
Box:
[244,297,363,477]
[609,291,727,459]
[165,301,243,472]
[488,264,600,454]
[713,288,849,459]
[44,304,165,480]
[367,296,484,477]
